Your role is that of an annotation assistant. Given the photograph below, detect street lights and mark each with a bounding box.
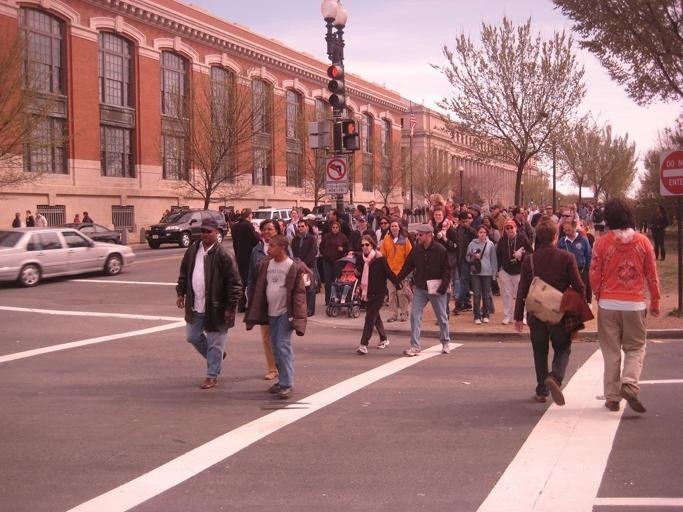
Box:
[520,180,561,212]
[319,0,349,217]
[457,166,465,205]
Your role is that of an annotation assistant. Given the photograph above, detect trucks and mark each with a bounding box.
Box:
[309,204,332,232]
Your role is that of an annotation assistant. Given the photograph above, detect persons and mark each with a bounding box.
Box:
[74,214,80,222]
[175,193,667,413]
[82,211,93,223]
[12,212,21,227]
[34,213,47,227]
[25,210,34,227]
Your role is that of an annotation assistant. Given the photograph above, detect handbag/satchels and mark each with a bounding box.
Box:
[469,259,482,274]
[524,275,566,326]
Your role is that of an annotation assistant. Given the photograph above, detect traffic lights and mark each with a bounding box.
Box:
[326,63,347,109]
[341,118,361,152]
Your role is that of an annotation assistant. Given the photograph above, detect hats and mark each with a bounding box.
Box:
[503,220,516,226]
[358,216,367,221]
[466,204,481,215]
[200,218,217,231]
[413,224,434,233]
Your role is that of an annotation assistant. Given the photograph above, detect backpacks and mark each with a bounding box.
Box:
[592,205,604,223]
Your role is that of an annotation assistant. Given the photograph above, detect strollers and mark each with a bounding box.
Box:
[324,250,364,318]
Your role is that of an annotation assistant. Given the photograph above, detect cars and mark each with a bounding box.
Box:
[0,225,137,289]
[56,219,123,247]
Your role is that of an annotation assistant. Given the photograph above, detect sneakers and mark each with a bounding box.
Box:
[404,348,420,356]
[604,399,619,411]
[265,370,292,399]
[451,304,527,325]
[387,317,407,322]
[377,339,389,349]
[201,378,217,388]
[619,384,646,413]
[543,375,565,405]
[442,345,449,352]
[357,344,367,353]
[535,393,548,402]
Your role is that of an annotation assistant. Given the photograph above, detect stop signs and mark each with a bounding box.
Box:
[661,150,682,195]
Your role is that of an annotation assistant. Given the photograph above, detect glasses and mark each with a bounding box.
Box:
[360,243,371,248]
[379,221,388,225]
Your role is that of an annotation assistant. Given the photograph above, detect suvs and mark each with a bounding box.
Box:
[249,206,295,237]
[143,207,230,250]
